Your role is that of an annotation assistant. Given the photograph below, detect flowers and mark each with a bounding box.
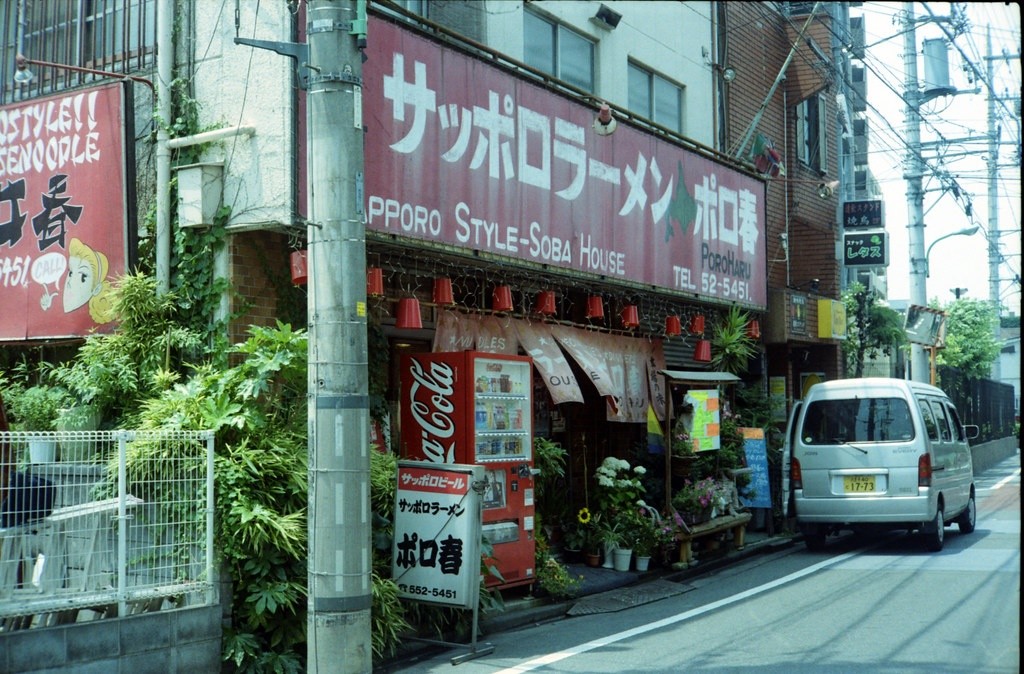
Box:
[530,395,745,606]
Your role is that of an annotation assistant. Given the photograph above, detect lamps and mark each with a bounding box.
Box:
[578,96,617,137]
[13,52,157,96]
[793,279,819,293]
[767,177,840,199]
[702,45,736,81]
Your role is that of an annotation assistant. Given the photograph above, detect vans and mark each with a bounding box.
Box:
[781,377,980,552]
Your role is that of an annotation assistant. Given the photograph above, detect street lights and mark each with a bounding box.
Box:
[907,226,979,385]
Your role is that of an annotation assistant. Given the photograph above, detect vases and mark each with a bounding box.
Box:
[672,455,699,477]
[551,526,565,544]
[613,549,632,571]
[587,553,601,568]
[635,555,651,571]
[602,547,614,568]
[564,546,582,564]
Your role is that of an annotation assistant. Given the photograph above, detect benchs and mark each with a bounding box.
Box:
[675,512,753,563]
